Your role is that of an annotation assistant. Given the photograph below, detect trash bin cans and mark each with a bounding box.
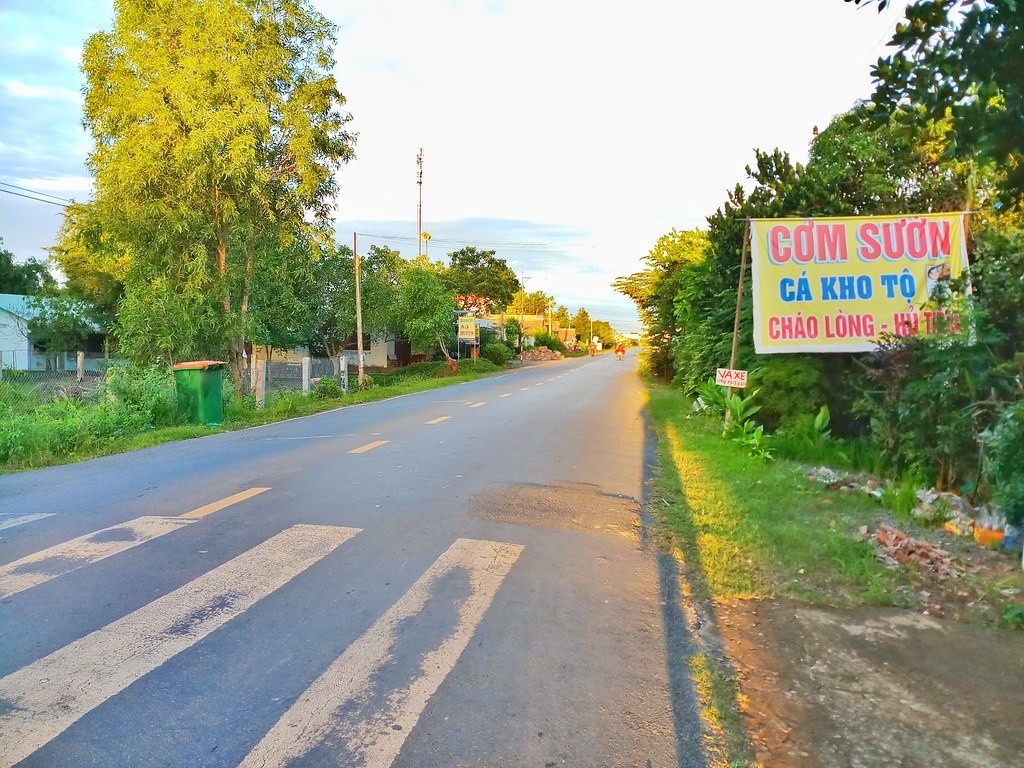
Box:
[171,359,228,426]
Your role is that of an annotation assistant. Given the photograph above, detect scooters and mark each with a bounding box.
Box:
[589,345,597,357]
[615,345,625,360]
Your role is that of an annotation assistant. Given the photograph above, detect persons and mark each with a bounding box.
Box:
[588,342,596,354]
[615,342,626,357]
